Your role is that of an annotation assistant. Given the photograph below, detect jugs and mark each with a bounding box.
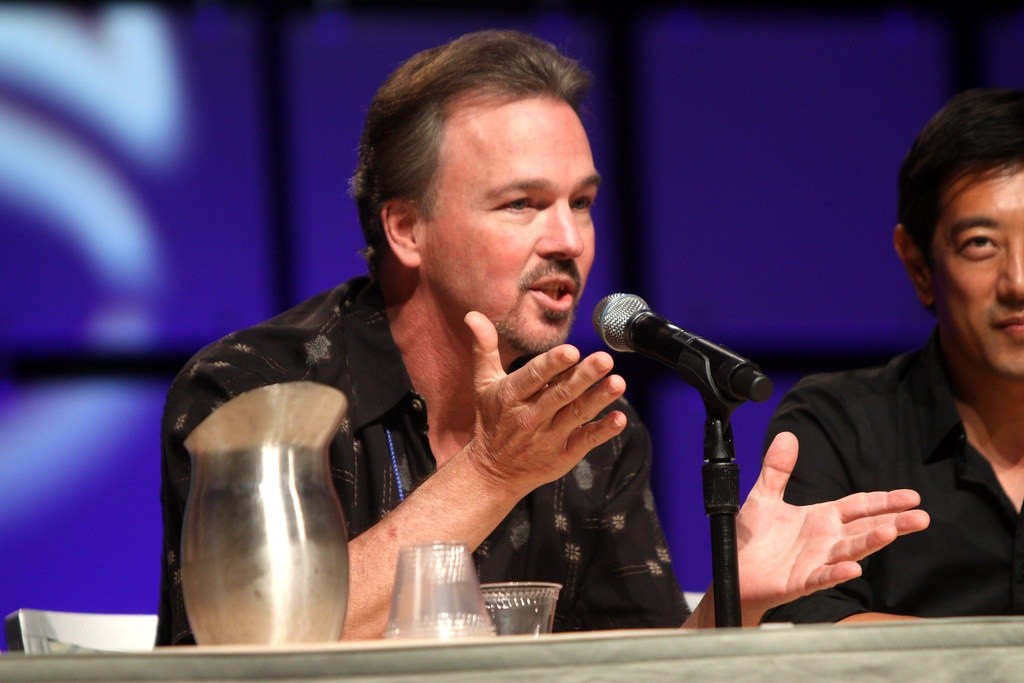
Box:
[182,381,349,648]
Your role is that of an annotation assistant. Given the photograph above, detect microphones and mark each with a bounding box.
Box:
[591,291,777,404]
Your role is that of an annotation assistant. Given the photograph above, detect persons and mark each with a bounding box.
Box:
[769,86,1023,625]
[156,32,929,650]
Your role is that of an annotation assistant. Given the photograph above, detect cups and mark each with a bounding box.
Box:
[480,582,562,636]
[379,543,498,639]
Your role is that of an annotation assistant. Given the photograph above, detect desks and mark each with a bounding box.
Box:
[0,615,1024,683]
